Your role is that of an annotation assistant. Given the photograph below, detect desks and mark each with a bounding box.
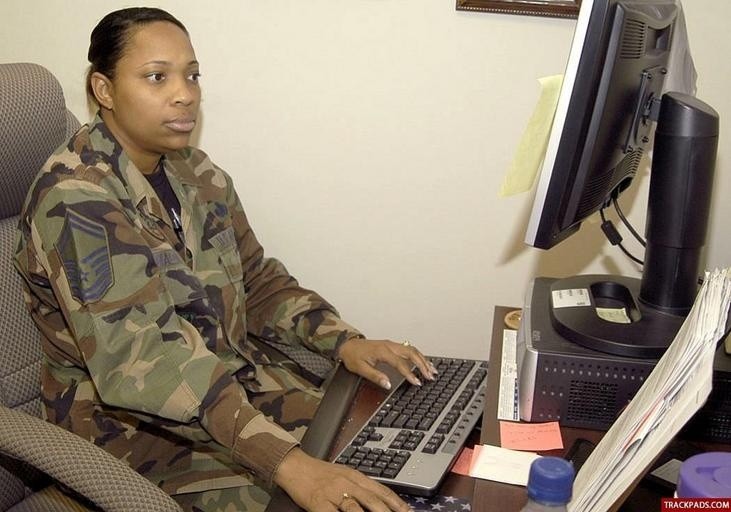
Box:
[265,305,731,512]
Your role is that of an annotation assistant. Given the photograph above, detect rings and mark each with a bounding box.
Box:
[338,493,352,506]
[402,340,410,347]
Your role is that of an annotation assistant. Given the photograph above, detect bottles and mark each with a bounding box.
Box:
[677,450,731,512]
[516,456,575,511]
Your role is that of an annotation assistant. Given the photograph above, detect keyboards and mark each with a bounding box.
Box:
[331,355,489,497]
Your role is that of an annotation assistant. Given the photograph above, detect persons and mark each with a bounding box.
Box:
[11,7,438,512]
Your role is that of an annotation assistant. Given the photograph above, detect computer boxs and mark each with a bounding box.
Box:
[516,277,731,445]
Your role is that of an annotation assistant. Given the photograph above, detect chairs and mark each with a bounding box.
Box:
[0,63,335,512]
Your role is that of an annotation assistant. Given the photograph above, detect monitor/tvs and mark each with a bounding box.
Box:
[522,0,731,358]
